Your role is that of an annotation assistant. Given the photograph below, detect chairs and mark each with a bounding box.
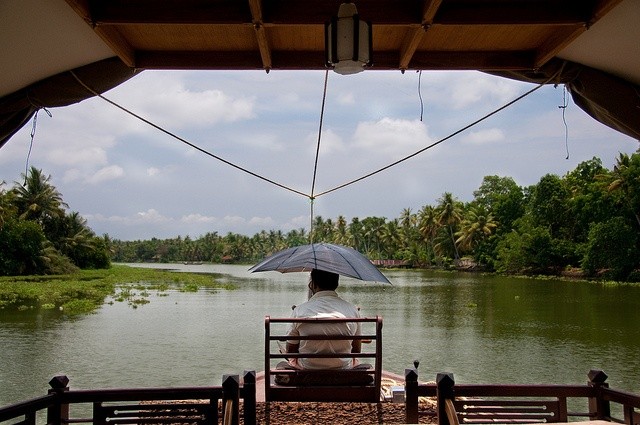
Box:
[263,316,383,425]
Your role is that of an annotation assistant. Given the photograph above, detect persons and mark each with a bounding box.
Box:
[283,264,364,370]
